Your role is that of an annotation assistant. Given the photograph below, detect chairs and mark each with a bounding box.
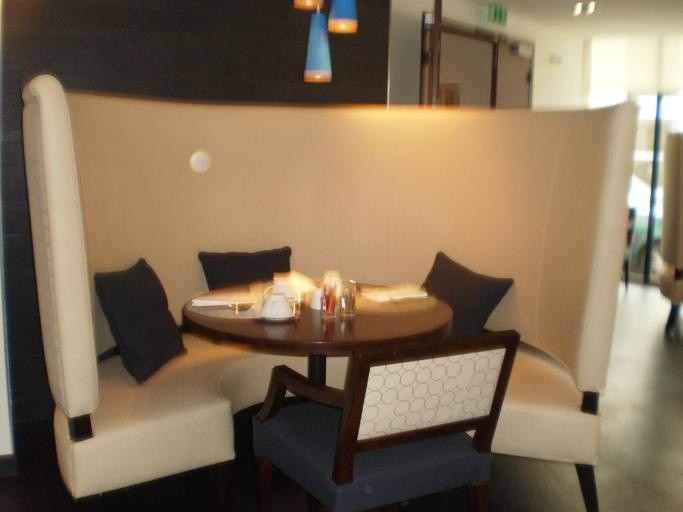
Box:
[624,206,638,289]
[252,329,520,512]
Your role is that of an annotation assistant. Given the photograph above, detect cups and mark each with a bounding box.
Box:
[339,279,357,318]
[309,287,320,311]
[319,280,336,319]
[262,292,296,319]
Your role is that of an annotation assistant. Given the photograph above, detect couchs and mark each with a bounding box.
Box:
[18,75,640,499]
[658,133,683,335]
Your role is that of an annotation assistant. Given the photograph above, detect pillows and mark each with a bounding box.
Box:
[421,248,514,334]
[197,248,292,342]
[94,255,188,383]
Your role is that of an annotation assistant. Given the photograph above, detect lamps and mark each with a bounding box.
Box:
[291,1,357,83]
